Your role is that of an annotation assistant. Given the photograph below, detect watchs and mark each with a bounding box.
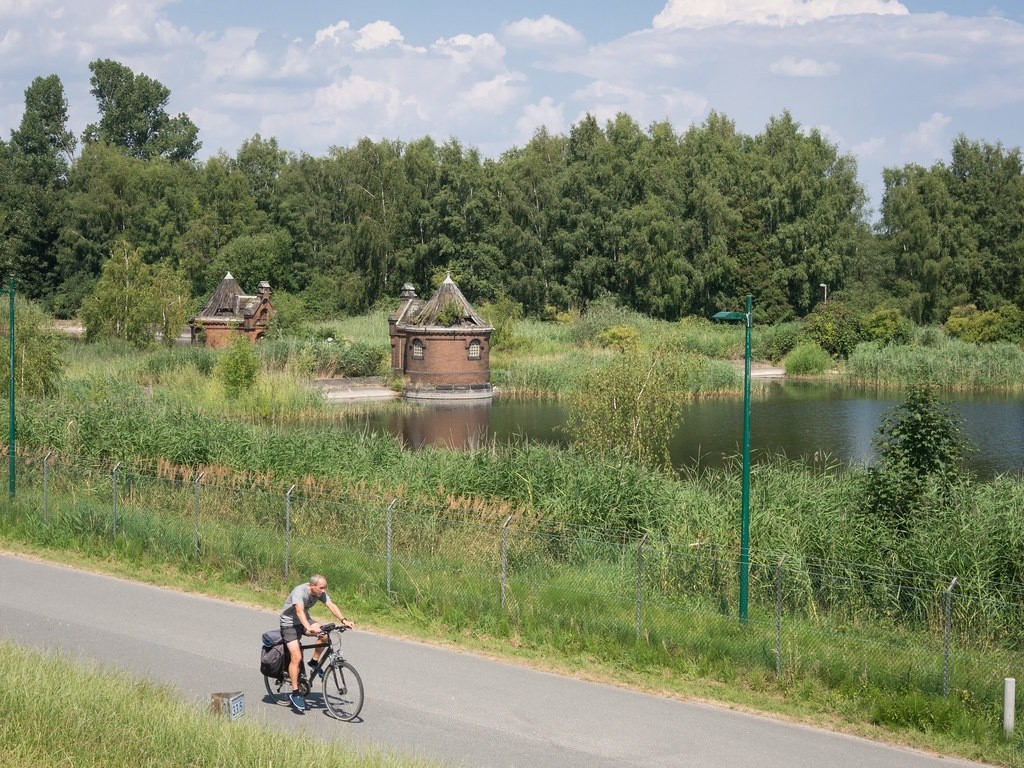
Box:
[341,618,347,624]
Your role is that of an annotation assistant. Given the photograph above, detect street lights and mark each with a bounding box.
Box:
[711,290,758,627]
[819,283,829,304]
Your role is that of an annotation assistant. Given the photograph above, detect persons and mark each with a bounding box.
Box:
[280,573,354,710]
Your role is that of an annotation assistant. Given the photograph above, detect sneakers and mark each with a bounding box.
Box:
[307,660,324,677]
[290,692,305,709]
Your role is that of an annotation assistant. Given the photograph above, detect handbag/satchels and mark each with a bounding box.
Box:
[260,644,285,679]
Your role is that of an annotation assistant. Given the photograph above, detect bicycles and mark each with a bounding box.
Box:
[260,623,365,721]
